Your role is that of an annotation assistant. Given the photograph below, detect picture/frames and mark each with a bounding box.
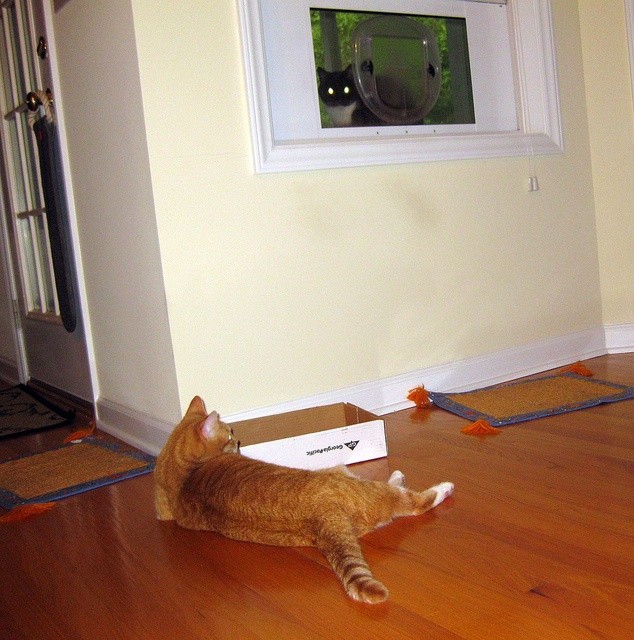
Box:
[235,0,566,176]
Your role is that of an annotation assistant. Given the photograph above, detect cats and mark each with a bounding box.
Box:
[153,396,455,605]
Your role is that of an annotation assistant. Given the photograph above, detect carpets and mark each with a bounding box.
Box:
[0,378,76,437]
[409,369,634,432]
[0,437,165,509]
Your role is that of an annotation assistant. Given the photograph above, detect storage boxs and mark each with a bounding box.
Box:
[203,398,388,479]
